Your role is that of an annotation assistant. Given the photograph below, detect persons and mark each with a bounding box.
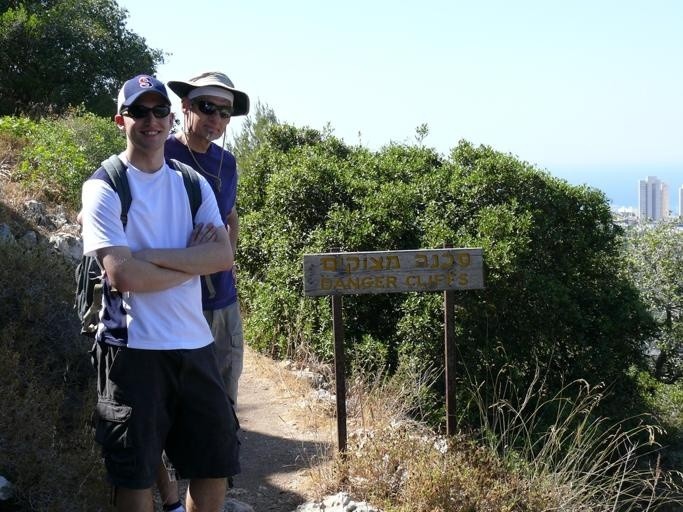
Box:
[77,74,242,512]
[151,71,249,512]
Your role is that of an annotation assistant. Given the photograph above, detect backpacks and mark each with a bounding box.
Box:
[72,256,102,338]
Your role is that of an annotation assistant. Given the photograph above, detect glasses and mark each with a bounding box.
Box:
[193,100,234,118]
[127,105,170,119]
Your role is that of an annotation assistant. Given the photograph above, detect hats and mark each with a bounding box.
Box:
[167,72,249,116]
[117,74,171,115]
